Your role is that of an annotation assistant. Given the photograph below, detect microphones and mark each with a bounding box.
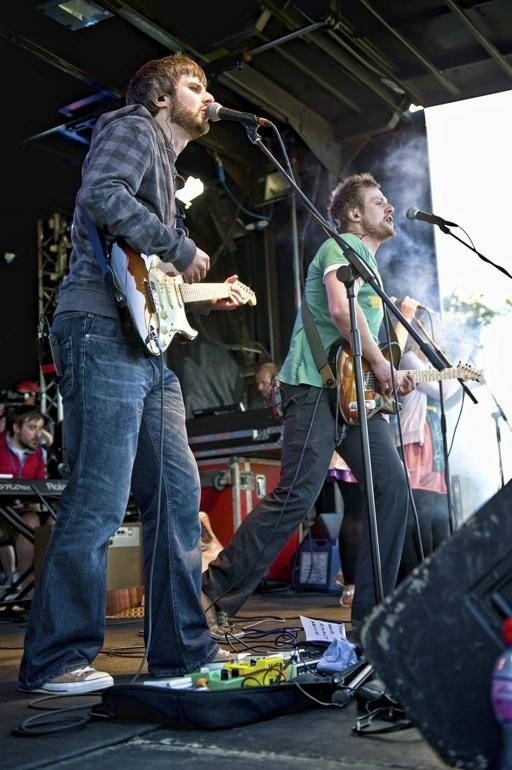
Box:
[205,102,268,126]
[389,296,424,309]
[406,206,458,227]
[38,437,56,460]
[453,335,484,349]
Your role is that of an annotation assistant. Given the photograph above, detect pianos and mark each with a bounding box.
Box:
[0,475,71,514]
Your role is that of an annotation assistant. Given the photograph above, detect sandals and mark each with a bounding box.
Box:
[334,569,356,609]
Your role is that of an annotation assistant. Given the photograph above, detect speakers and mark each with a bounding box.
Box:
[357,479,512,770]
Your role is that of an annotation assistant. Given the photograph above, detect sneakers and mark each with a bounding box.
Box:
[199,646,252,662]
[18,665,113,696]
[201,590,246,641]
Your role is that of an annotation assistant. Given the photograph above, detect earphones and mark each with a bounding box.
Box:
[157,94,166,102]
[354,213,359,218]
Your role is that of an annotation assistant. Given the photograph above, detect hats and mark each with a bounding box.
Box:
[18,381,38,392]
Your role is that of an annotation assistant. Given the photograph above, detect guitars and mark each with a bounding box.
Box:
[327,336,487,428]
[107,234,258,356]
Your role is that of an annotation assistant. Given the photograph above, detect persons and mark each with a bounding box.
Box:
[0,381,55,447]
[12,53,253,696]
[397,308,475,590]
[194,174,418,641]
[0,404,47,602]
[327,452,366,607]
[256,362,282,403]
